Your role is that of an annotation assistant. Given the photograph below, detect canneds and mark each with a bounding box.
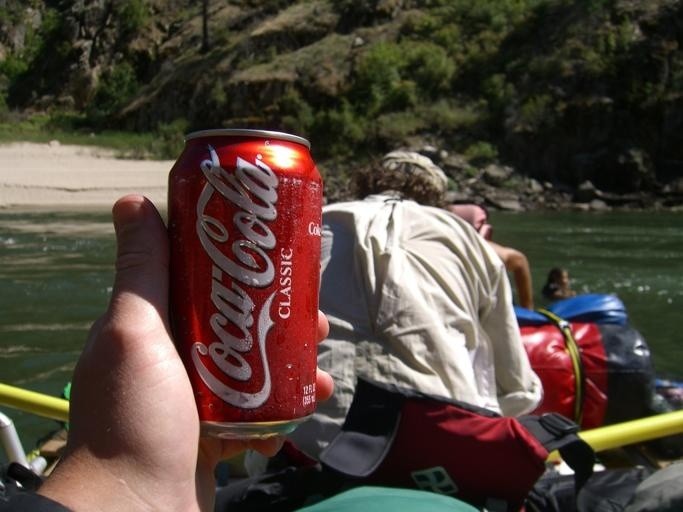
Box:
[168,128,323,440]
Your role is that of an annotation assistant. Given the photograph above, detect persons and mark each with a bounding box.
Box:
[541,266,576,301]
[0,190,334,512]
[284,147,544,493]
[444,198,535,317]
